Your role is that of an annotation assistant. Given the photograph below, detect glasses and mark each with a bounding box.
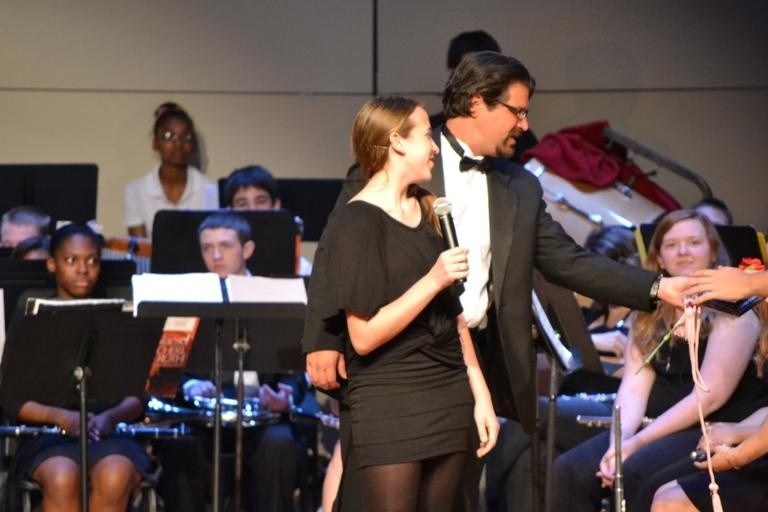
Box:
[496,101,528,119]
[156,131,193,141]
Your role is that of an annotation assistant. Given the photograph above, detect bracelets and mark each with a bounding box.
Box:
[726,447,739,470]
[649,273,664,300]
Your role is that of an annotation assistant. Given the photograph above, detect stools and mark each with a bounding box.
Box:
[4,429,158,512]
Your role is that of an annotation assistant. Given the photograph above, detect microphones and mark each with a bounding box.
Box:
[432,196,468,287]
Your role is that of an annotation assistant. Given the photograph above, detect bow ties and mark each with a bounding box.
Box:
[459,157,492,174]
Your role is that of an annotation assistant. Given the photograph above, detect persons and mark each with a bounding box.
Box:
[550,208,768,511]
[320,95,503,512]
[428,28,539,165]
[0,222,152,511]
[124,104,221,238]
[219,166,314,277]
[151,213,310,511]
[2,206,56,275]
[481,196,767,512]
[300,50,694,512]
